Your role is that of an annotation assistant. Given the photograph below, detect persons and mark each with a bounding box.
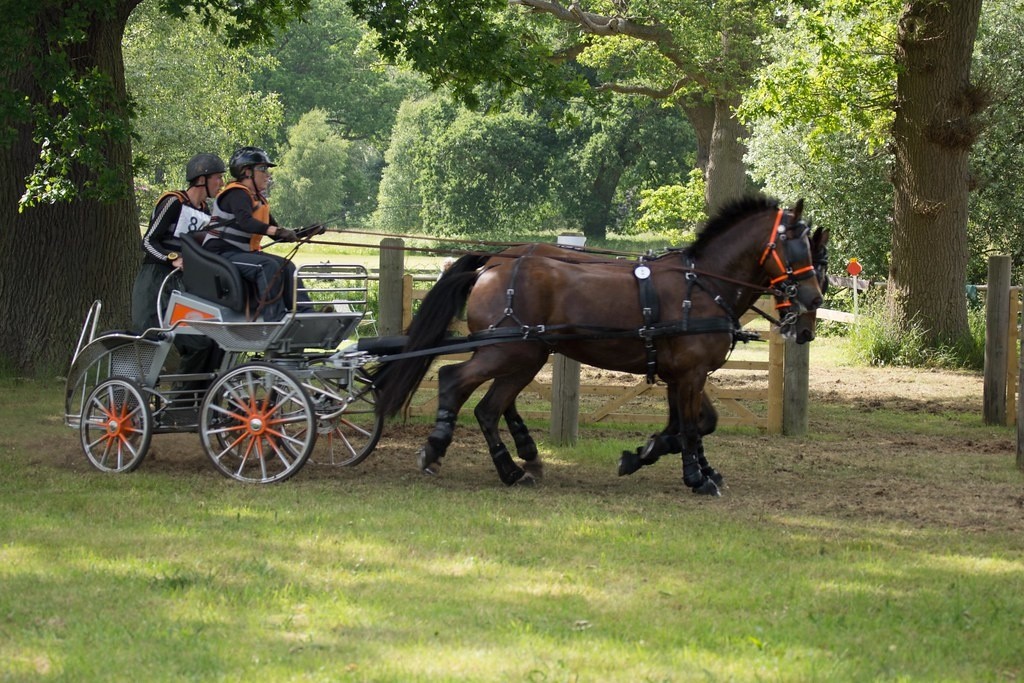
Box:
[141,152,226,271]
[202,147,333,322]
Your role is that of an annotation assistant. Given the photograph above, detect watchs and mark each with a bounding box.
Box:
[167,252,178,268]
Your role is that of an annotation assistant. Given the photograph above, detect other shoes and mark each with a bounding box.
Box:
[308,305,333,313]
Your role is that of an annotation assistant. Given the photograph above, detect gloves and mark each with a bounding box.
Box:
[299,223,326,237]
[274,227,297,243]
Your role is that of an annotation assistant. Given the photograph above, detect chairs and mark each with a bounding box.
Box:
[180,229,248,314]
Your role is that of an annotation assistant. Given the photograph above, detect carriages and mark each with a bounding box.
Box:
[59,193,832,504]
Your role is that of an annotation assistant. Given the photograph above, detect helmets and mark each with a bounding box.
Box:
[229,147,277,178]
[186,153,227,182]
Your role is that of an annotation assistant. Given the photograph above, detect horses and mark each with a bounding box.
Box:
[360,190,830,497]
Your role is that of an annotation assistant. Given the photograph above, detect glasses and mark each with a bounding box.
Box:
[255,166,268,172]
